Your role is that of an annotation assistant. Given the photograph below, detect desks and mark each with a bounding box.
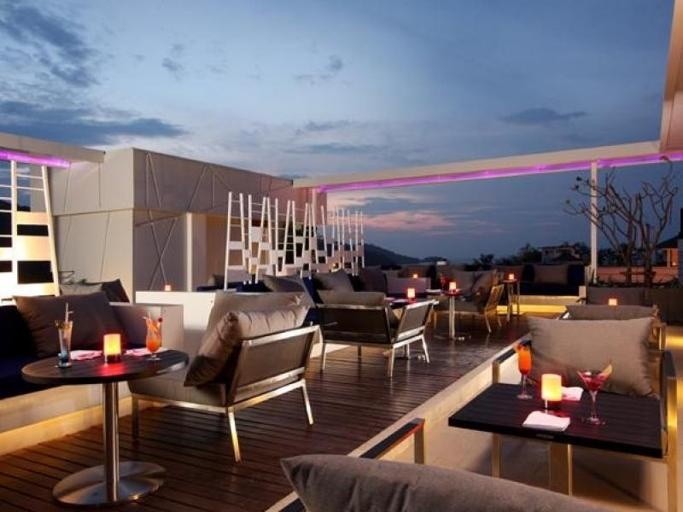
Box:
[22,347,188,506]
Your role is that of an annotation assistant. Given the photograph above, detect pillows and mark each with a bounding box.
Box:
[61,283,103,299]
[184,301,311,388]
[199,288,305,353]
[79,280,128,301]
[15,290,125,356]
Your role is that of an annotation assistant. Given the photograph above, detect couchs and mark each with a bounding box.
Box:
[1,303,183,455]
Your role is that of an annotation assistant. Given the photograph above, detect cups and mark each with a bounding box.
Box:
[56,319,73,366]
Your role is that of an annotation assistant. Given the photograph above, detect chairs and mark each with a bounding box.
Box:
[129,325,320,460]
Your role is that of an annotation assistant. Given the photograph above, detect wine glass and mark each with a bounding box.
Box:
[516,345,533,400]
[577,370,610,425]
[145,320,162,361]
